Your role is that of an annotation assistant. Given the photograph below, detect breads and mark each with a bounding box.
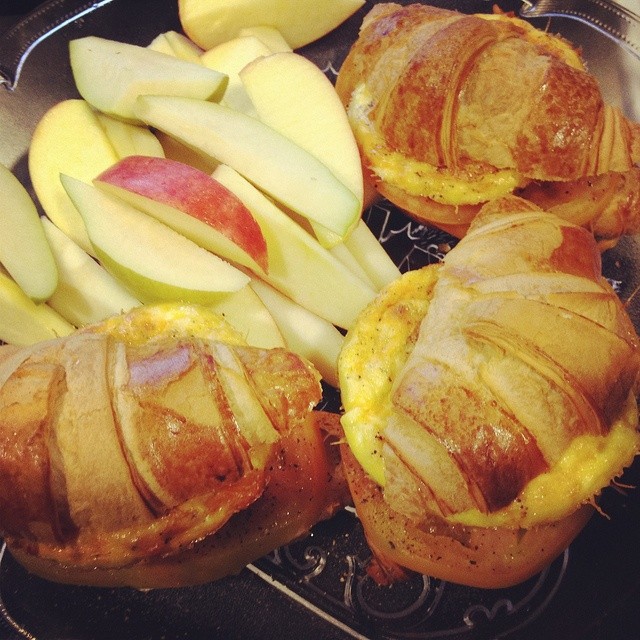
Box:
[332,1,640,241]
[337,194,640,590]
[0,300,350,589]
